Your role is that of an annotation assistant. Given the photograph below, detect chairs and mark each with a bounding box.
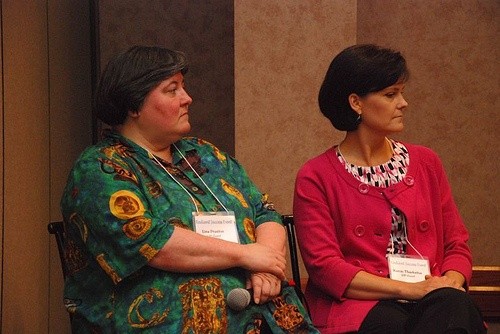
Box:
[279,211,312,317]
[44,221,102,333]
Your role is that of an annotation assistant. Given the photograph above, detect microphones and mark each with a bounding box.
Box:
[226,280,297,312]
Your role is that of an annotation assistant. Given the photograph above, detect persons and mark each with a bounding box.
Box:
[291,45,489,334]
[61,43,320,334]
